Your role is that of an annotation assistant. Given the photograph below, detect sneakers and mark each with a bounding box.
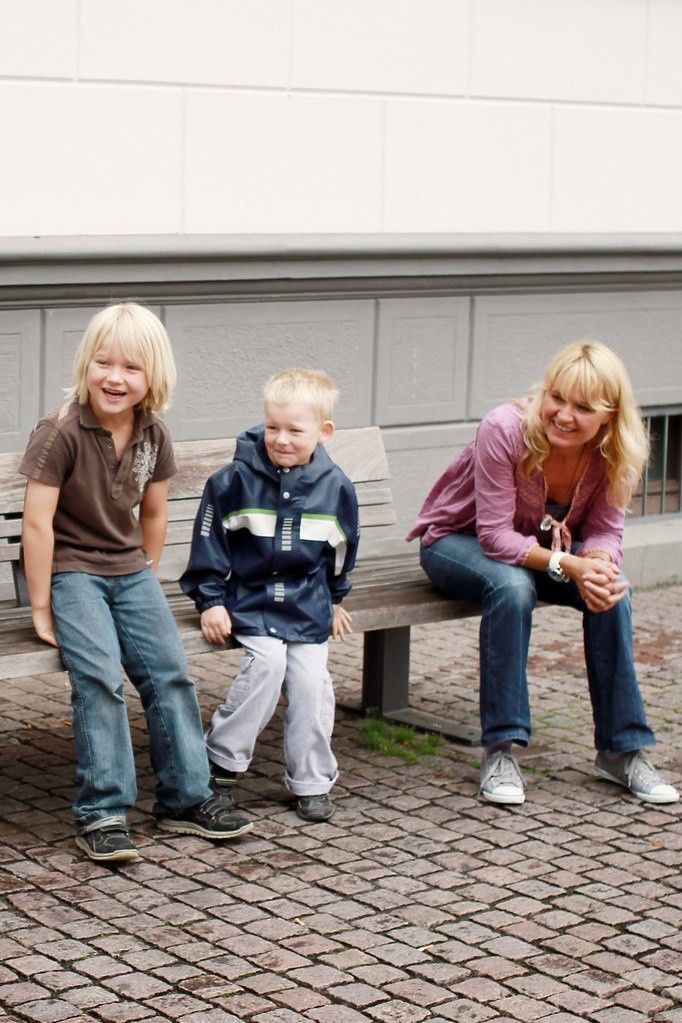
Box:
[157,797,255,840]
[594,750,679,804]
[297,793,336,820]
[203,727,237,807]
[477,750,527,804]
[75,824,139,862]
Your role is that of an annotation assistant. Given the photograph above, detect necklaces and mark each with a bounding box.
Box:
[557,443,586,518]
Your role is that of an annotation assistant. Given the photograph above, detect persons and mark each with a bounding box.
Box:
[18,300,254,863]
[178,369,358,822]
[410,338,681,804]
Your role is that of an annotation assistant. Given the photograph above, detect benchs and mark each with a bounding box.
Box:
[0,426,551,748]
